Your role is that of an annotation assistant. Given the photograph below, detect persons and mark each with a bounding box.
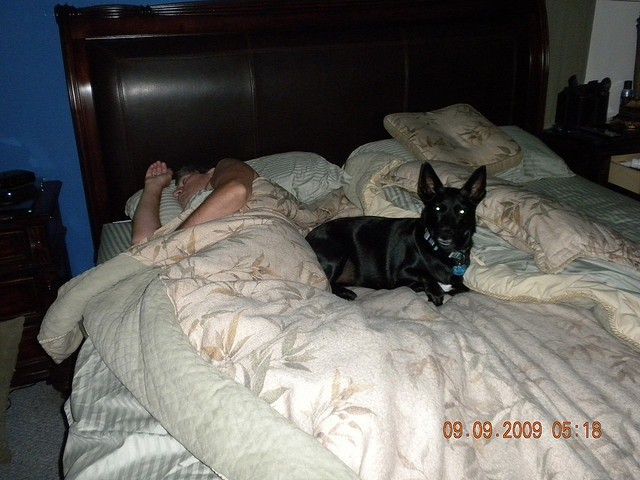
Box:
[130,158,261,245]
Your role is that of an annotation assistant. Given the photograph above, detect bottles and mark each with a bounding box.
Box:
[618,80,633,113]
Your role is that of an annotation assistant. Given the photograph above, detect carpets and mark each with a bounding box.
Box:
[1,314,85,479]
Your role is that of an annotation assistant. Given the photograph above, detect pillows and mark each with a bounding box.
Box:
[339,125,577,210]
[123,151,353,227]
[384,103,524,188]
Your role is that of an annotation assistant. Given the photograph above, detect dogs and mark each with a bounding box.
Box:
[304,161,488,307]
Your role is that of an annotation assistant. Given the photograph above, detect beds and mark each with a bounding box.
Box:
[37,3,638,480]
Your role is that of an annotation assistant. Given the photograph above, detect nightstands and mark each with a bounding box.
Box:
[540,127,585,168]
[0,180,74,324]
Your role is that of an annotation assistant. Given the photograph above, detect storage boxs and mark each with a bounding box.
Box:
[609,153,640,195]
